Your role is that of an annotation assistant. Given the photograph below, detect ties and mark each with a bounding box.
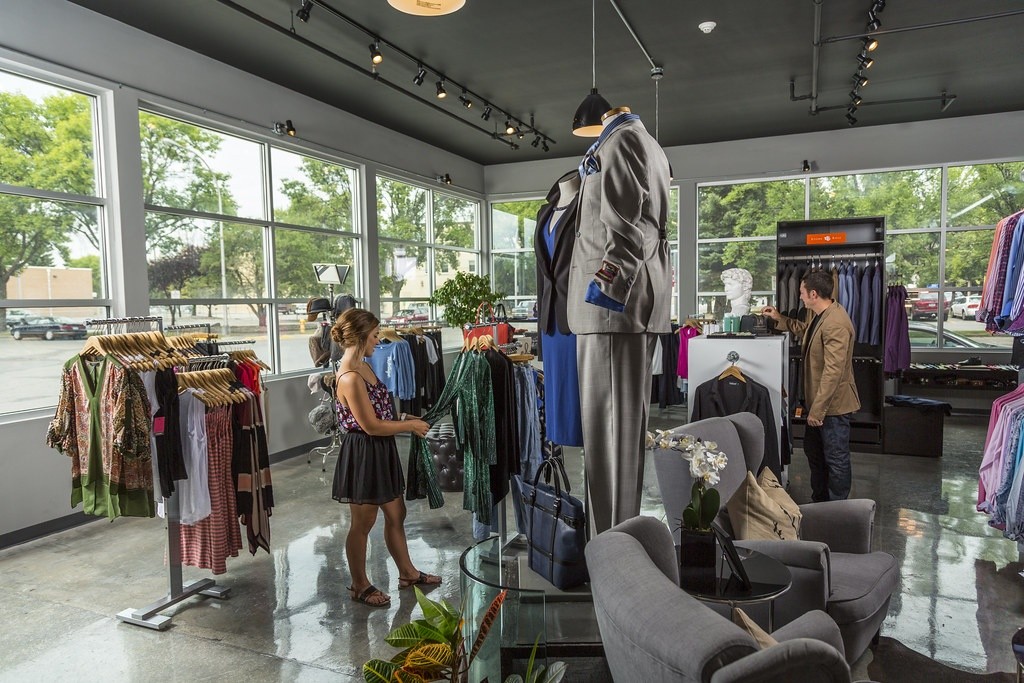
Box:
[578,141,600,179]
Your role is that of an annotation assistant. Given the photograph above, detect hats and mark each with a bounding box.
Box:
[306,293,355,434]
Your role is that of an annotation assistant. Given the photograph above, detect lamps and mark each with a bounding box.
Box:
[273,0,557,185]
[571,0,612,138]
[802,0,893,172]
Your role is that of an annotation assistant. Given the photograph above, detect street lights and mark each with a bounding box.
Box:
[161,137,231,335]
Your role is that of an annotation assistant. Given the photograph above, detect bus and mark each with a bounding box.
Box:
[502,295,537,317]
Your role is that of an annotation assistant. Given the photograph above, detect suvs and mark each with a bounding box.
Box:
[904,292,950,322]
[512,301,538,319]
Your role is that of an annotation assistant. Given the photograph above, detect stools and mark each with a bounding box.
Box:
[885,403,944,457]
[426,424,464,493]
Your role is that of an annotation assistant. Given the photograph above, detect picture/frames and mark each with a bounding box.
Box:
[710,520,752,589]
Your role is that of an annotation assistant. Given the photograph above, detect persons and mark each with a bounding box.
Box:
[760,271,862,502]
[721,268,752,316]
[329,309,442,606]
[534,108,673,538]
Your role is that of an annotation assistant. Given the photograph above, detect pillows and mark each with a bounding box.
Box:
[728,466,803,650]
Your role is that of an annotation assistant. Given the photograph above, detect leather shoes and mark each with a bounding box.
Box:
[958,356,981,365]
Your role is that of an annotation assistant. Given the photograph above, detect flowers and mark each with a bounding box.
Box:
[640,427,731,526]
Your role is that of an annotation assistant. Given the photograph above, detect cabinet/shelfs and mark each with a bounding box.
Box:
[307,264,361,471]
[776,214,887,454]
[688,333,789,489]
[901,362,1018,416]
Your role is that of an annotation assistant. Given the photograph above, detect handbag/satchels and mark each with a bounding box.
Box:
[740,314,772,336]
[521,456,589,591]
[463,300,515,350]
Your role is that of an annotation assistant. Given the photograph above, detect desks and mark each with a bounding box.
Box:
[671,544,791,637]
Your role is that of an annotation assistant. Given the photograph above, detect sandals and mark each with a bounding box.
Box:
[350,584,390,607]
[398,571,442,590]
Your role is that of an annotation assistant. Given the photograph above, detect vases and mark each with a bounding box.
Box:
[676,518,720,571]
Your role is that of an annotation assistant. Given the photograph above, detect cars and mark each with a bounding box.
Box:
[381,308,429,328]
[10,316,88,341]
[951,295,982,320]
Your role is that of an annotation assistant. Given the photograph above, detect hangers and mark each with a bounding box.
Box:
[700,356,768,394]
[664,315,717,343]
[75,314,538,407]
[775,253,883,280]
[992,382,1024,427]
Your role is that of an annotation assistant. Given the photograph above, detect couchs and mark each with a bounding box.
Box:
[653,413,900,666]
[585,516,882,683]
